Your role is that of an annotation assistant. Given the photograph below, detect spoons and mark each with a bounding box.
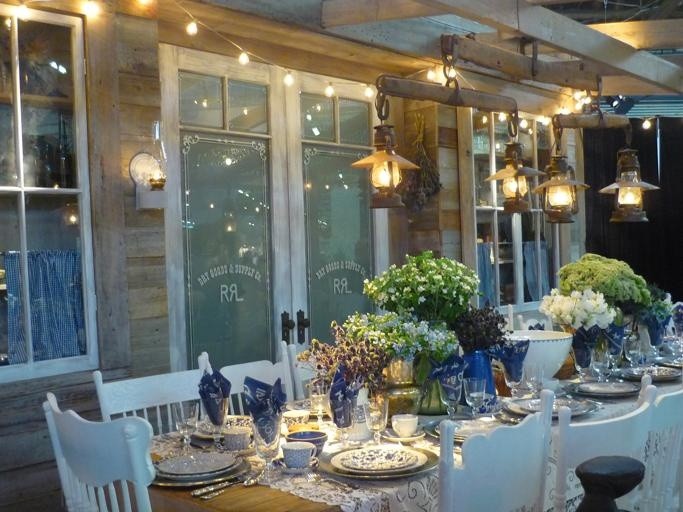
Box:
[198,470,262,500]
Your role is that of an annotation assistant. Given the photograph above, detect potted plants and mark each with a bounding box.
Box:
[556,252,652,382]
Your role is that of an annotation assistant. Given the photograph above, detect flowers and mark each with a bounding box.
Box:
[362,250,486,408]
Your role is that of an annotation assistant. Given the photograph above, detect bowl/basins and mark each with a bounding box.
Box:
[516,331,576,383]
[284,430,327,455]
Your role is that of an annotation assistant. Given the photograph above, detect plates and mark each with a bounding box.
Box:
[150,451,247,489]
[271,457,318,474]
[320,366,682,478]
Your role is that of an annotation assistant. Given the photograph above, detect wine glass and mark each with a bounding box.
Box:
[309,335,683,441]
[171,397,281,486]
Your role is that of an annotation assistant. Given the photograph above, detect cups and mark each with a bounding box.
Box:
[224,426,250,452]
[280,439,315,467]
[390,410,416,437]
[282,409,308,431]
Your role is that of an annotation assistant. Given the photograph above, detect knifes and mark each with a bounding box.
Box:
[190,469,255,497]
